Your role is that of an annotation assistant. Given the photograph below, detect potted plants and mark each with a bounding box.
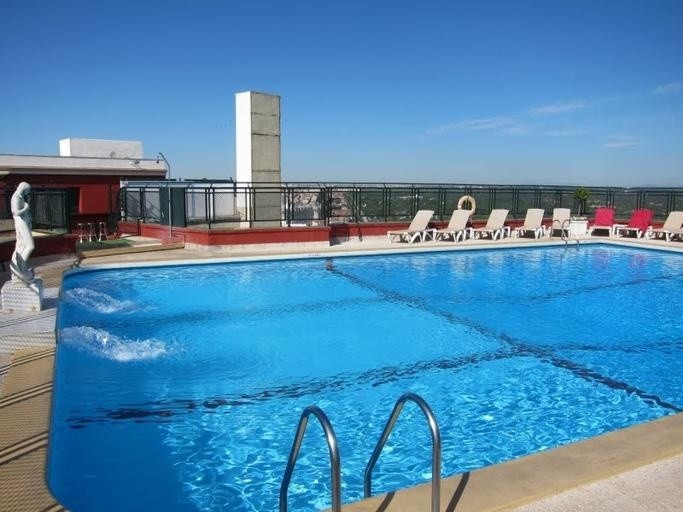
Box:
[569,188,590,238]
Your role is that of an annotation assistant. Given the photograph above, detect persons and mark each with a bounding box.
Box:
[11,181,36,280]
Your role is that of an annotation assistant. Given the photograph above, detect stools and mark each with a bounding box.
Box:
[76,222,108,243]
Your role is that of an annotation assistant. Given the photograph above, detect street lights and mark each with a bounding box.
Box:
[157,152,171,239]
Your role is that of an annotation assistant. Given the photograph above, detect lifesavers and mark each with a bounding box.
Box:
[457,195,475,214]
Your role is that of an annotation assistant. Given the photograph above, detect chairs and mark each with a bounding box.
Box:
[387,210,436,245]
[434,210,475,243]
[550,207,571,238]
[516,209,546,240]
[671,229,682,242]
[615,209,655,239]
[587,208,614,236]
[474,209,512,240]
[652,211,682,242]
